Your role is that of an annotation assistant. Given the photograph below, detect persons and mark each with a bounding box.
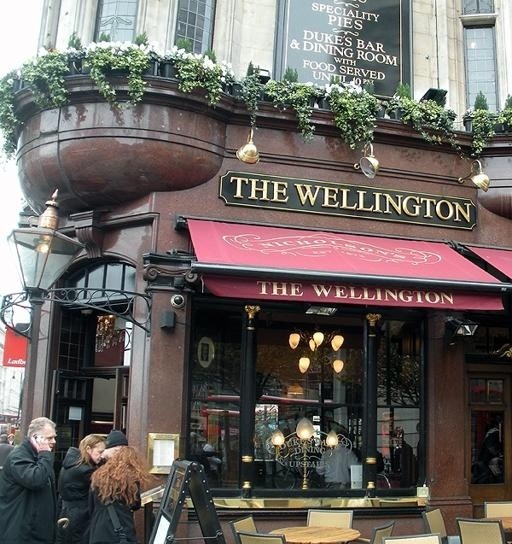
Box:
[0,417,159,544]
[316,424,358,484]
[392,426,413,488]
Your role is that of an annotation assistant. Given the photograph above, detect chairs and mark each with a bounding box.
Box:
[230,514,259,544]
[484,501,512,517]
[350,520,393,544]
[237,531,286,544]
[422,508,461,544]
[455,517,512,544]
[382,534,443,544]
[306,508,355,527]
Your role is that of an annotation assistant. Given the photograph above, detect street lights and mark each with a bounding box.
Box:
[289,332,344,444]
[0,184,86,445]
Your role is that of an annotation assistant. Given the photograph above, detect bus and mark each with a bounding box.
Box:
[0,414,19,434]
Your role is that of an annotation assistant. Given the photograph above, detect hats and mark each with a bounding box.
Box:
[105,430,128,448]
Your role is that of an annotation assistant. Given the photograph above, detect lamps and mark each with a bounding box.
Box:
[355,141,381,180]
[95,314,125,353]
[257,69,271,83]
[235,126,260,163]
[287,324,345,375]
[439,316,478,349]
[459,160,490,192]
[272,417,339,489]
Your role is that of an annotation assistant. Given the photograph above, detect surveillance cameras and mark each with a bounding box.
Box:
[171,294,185,308]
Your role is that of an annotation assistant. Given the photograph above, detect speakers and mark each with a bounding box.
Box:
[161,311,176,330]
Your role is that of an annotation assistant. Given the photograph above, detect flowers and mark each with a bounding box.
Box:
[0,32,512,164]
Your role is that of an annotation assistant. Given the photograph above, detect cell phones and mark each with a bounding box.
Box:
[32,433,42,443]
